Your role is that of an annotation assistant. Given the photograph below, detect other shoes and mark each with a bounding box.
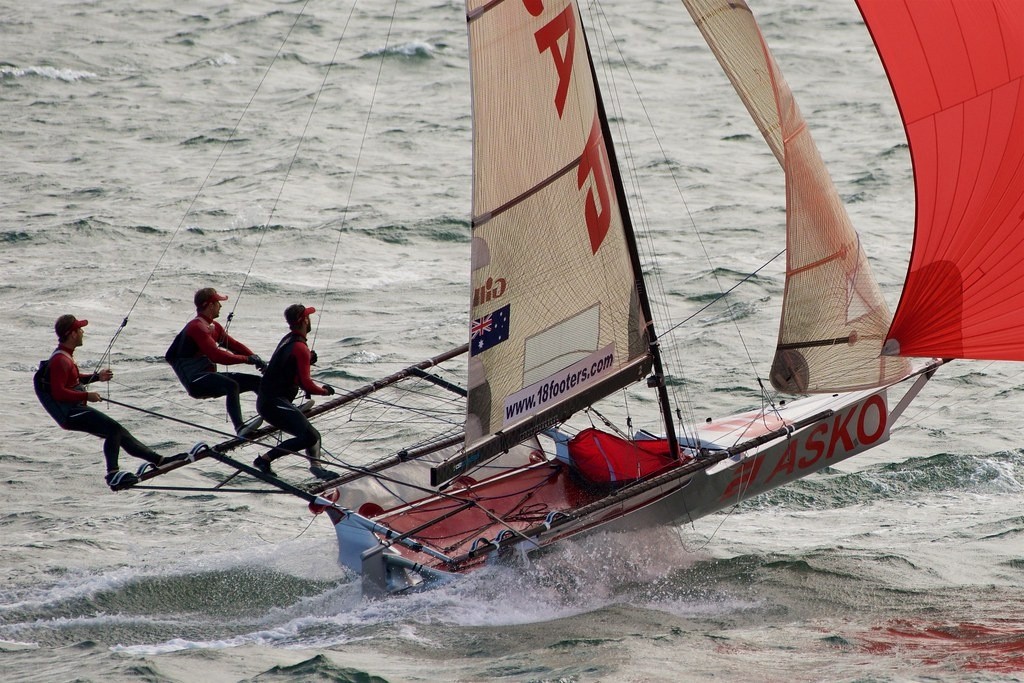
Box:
[297,399,314,412]
[105,471,133,486]
[160,452,188,465]
[238,417,263,437]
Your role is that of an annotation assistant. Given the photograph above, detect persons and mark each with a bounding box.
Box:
[33,314,169,484]
[164,288,314,436]
[254,305,341,479]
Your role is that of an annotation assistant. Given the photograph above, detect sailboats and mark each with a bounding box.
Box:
[76,0,1024,598]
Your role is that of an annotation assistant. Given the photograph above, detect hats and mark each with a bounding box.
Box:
[196,291,229,306]
[59,318,88,338]
[288,307,315,325]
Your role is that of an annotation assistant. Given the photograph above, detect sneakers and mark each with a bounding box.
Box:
[310,465,340,480]
[253,455,277,477]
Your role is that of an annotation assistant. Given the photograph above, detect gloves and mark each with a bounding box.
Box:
[322,383,335,394]
[256,360,268,375]
[246,354,261,368]
[310,350,318,365]
